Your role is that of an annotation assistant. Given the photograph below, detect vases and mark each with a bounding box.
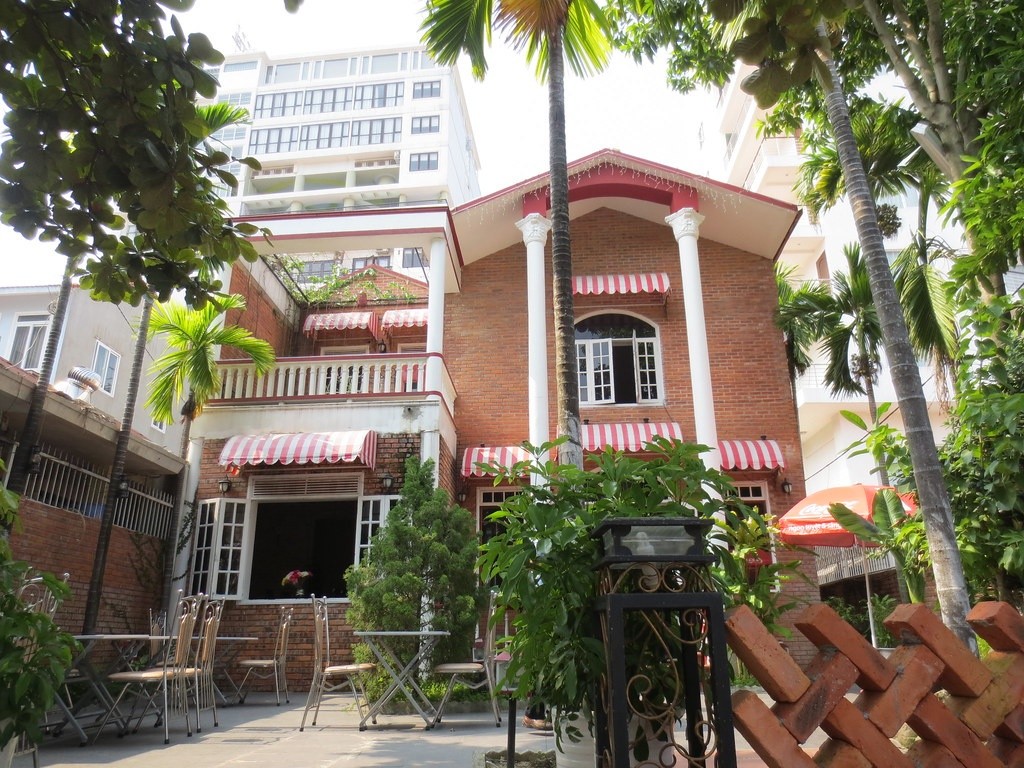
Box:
[295,587,306,598]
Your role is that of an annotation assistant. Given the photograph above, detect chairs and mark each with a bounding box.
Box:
[90,588,204,746]
[133,594,226,736]
[229,606,294,708]
[148,606,200,708]
[9,565,70,768]
[430,590,502,729]
[299,594,378,732]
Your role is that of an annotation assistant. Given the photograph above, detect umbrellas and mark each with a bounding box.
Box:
[778,485,921,650]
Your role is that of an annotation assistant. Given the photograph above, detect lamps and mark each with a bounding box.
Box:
[378,338,387,353]
[457,486,468,505]
[379,470,395,492]
[781,477,794,497]
[115,472,130,498]
[218,473,232,495]
[26,445,43,476]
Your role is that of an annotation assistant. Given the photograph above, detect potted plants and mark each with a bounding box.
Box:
[469,433,768,768]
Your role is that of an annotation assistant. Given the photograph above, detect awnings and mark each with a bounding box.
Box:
[217,430,377,472]
[461,446,531,477]
[571,272,671,296]
[303,312,378,341]
[718,440,784,471]
[554,422,683,462]
[381,309,428,337]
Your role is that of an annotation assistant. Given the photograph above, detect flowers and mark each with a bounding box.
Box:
[280,570,312,590]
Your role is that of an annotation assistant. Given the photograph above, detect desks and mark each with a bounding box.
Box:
[352,631,451,732]
[166,637,259,707]
[50,635,166,748]
